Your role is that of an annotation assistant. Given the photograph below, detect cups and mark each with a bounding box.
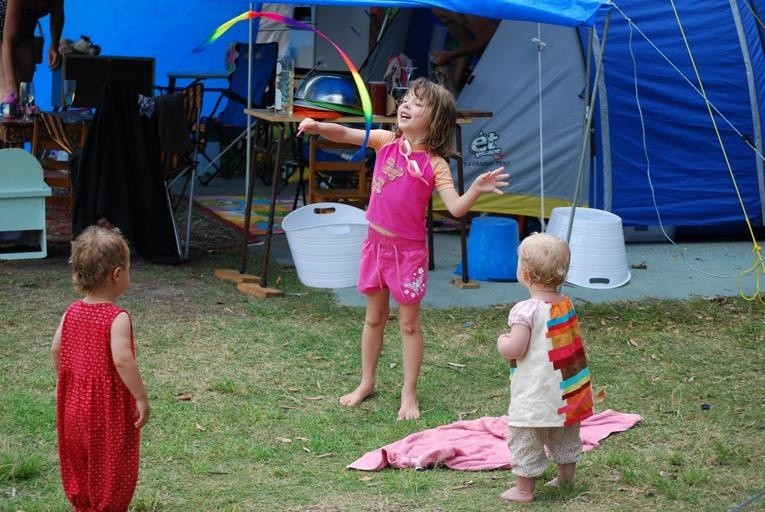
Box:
[368,81,408,118]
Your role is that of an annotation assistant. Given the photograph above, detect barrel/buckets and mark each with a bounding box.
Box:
[457,214,519,281]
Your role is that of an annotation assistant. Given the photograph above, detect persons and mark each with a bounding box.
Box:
[426,7,498,88]
[0,0,64,99]
[49,224,151,512]
[497,230,593,505]
[296,75,510,422]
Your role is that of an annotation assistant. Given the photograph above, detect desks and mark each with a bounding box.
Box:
[239,109,494,289]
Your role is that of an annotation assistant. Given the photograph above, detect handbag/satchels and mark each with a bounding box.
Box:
[33,19,46,65]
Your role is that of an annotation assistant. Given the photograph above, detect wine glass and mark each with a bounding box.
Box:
[18,81,35,121]
[62,77,77,115]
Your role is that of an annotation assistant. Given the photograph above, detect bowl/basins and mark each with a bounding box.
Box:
[294,75,357,106]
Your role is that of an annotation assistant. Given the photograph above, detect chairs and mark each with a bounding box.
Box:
[1,146,53,260]
[308,127,370,216]
[31,81,206,264]
[165,42,280,186]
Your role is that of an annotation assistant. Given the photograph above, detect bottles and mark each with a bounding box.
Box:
[273,49,296,119]
[2,90,17,120]
